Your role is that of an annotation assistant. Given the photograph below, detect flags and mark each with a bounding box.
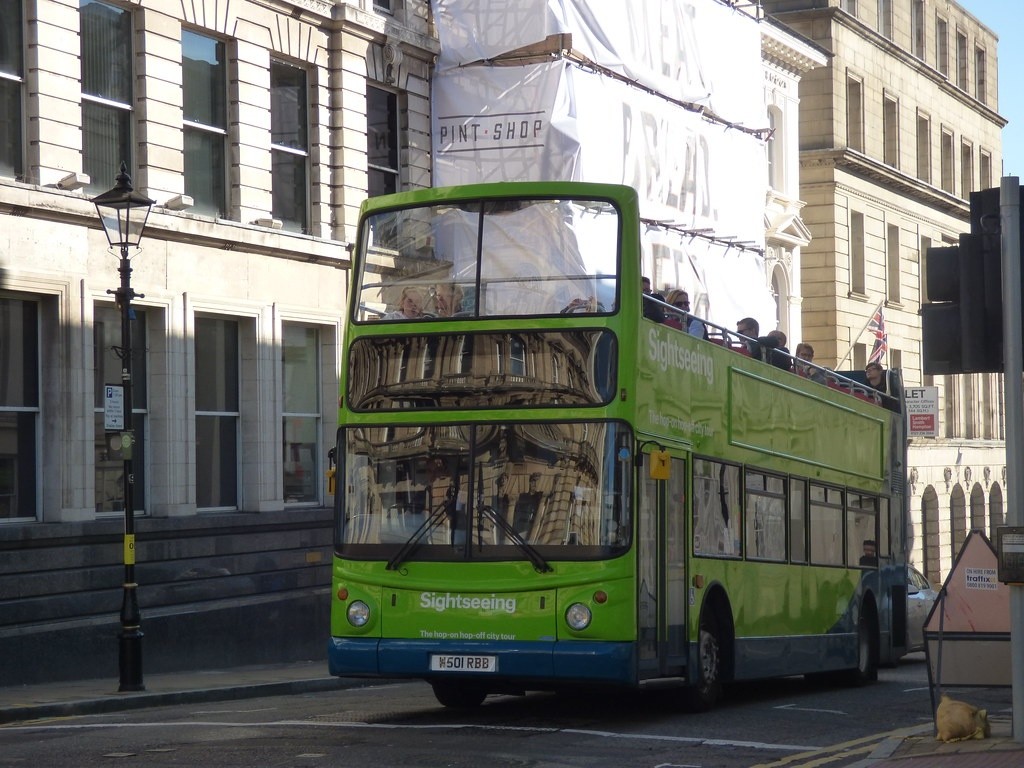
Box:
[866,306,887,364]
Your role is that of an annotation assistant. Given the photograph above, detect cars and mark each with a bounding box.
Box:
[907,564,940,654]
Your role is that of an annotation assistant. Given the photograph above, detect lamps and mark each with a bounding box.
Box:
[57,171,91,193]
[253,219,283,230]
[164,195,193,212]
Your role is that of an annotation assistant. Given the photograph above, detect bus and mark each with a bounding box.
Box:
[328,180,910,717]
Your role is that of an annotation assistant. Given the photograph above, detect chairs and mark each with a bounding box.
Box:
[790,364,807,378]
[827,375,840,391]
[839,382,852,394]
[847,388,868,401]
[708,333,732,349]
[863,393,882,407]
[731,341,752,358]
[664,312,683,330]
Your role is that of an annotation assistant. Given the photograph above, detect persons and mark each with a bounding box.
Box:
[860,362,901,415]
[381,287,439,319]
[558,280,606,314]
[497,472,509,498]
[641,276,710,341]
[737,317,768,361]
[528,472,543,495]
[767,331,792,371]
[791,344,834,384]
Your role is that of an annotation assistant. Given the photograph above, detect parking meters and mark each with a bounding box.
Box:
[996,526,1024,586]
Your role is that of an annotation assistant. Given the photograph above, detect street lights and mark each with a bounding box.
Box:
[93,163,156,688]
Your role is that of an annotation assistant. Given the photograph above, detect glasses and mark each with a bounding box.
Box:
[674,302,690,307]
[864,367,877,372]
[799,353,814,359]
[737,328,751,334]
[642,289,652,294]
